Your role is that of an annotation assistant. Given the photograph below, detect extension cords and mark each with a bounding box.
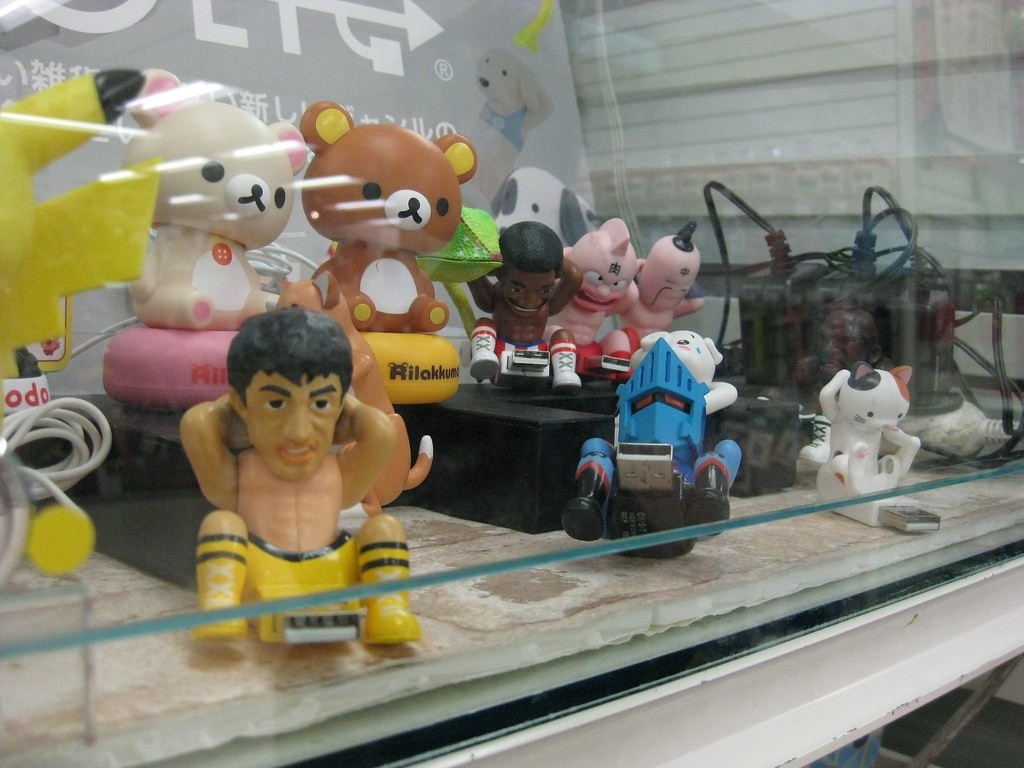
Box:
[802,398,989,463]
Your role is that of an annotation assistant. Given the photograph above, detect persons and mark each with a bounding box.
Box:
[180,308,424,647]
[470,221,581,391]
[623,222,705,385]
[547,217,636,383]
[748,302,893,489]
